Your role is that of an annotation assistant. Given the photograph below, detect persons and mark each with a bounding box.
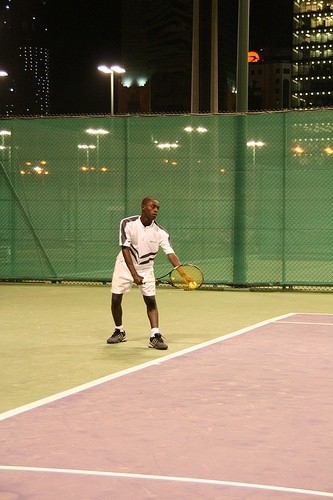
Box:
[107,196,196,350]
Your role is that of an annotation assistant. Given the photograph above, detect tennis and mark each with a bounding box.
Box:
[188,281,197,290]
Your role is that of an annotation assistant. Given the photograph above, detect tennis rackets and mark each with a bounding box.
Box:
[133,264,204,291]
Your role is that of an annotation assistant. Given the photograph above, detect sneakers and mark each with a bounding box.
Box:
[148,333,168,350]
[107,329,126,343]
